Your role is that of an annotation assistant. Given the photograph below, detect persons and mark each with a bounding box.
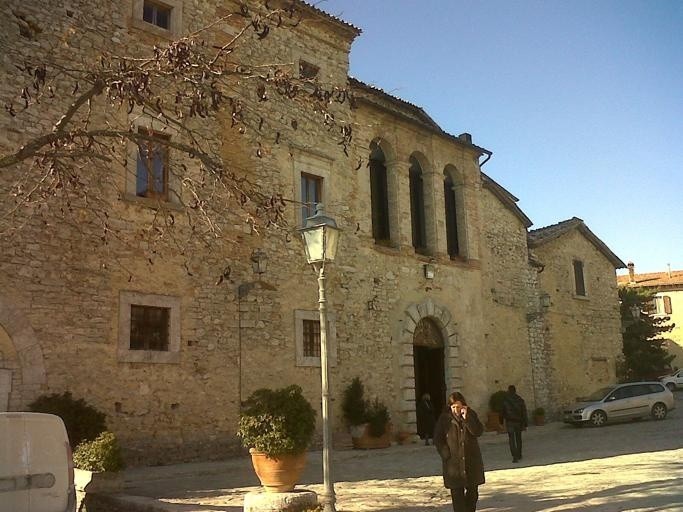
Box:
[417,393,436,445]
[498,385,529,463]
[431,391,486,512]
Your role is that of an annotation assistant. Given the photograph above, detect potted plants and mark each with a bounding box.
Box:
[488,391,509,430]
[72,432,126,493]
[340,377,394,450]
[532,408,546,427]
[234,383,318,493]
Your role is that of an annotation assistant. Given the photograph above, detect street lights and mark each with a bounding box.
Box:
[297,203,343,512]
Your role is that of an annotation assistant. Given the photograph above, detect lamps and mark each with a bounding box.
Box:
[239,249,278,300]
[526,294,552,324]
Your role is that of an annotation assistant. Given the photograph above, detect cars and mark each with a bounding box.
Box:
[561,382,675,427]
[658,367,683,391]
